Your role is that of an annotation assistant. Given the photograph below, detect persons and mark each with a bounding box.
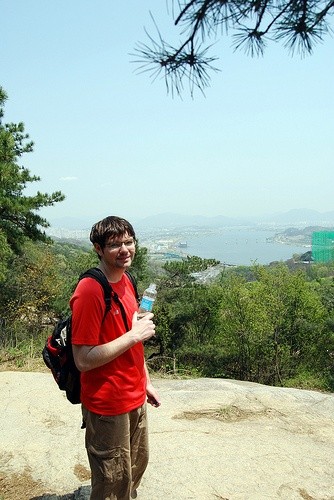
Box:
[69,215,161,500]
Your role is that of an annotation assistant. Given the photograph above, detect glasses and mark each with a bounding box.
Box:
[104,239,137,250]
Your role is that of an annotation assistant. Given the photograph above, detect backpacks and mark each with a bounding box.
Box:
[42,267,140,404]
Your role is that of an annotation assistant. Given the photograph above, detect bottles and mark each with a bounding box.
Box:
[137,282,157,320]
